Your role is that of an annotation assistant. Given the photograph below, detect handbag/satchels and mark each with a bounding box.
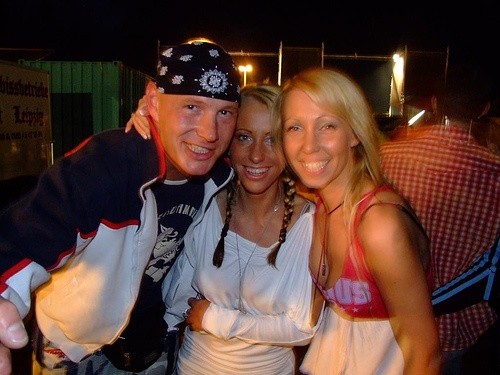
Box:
[102,298,167,372]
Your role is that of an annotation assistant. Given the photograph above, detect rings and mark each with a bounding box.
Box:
[182,309,191,319]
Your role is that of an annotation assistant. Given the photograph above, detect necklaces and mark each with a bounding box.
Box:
[318,192,344,275]
[235,187,282,316]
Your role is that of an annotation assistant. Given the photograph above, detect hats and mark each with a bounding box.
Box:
[155,42,242,106]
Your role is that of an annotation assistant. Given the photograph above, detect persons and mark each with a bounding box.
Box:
[0,41,242,375]
[371,62,500,375]
[271,68,444,375]
[125,84,326,375]
